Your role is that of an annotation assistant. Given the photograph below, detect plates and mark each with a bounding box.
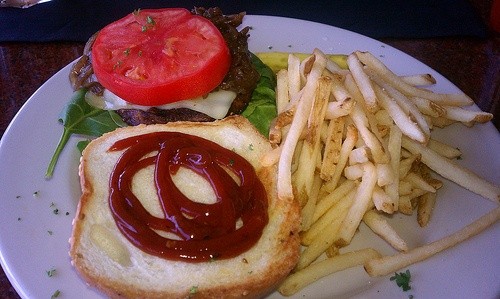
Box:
[1,15,499,299]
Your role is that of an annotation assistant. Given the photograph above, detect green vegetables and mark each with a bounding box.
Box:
[46,50,276,180]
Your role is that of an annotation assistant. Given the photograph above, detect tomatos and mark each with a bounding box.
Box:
[92,8,231,106]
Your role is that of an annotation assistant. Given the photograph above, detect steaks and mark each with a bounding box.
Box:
[67,6,260,125]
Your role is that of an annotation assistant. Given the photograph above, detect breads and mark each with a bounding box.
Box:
[67,113,300,299]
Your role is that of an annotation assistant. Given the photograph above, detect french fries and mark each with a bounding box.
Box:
[268,46,500,295]
